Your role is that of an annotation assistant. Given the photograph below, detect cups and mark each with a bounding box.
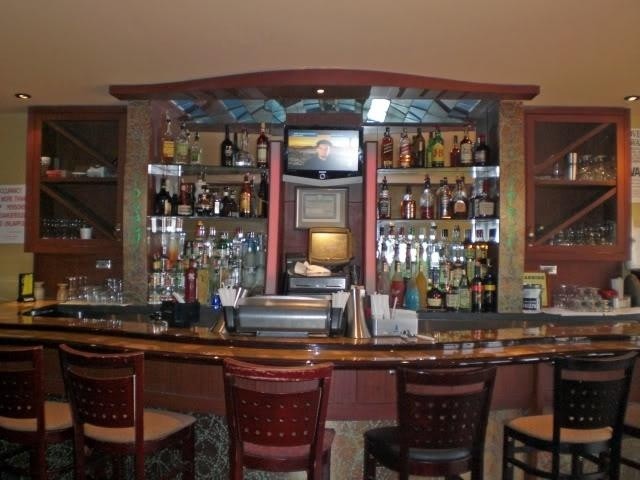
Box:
[79,223,94,240]
[78,289,115,305]
[40,216,83,240]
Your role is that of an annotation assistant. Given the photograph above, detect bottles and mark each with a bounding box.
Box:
[155,178,170,216]
[438,229,450,264]
[445,270,460,310]
[431,126,444,168]
[463,229,474,260]
[427,132,433,168]
[257,172,267,217]
[415,265,426,309]
[149,221,267,305]
[471,263,483,313]
[449,134,460,167]
[256,121,268,168]
[239,174,253,218]
[221,187,237,217]
[478,179,496,219]
[424,267,443,309]
[381,127,393,169]
[487,229,498,264]
[427,225,439,266]
[473,229,488,263]
[483,274,495,313]
[436,178,452,220]
[391,262,403,310]
[460,126,473,167]
[452,177,469,220]
[413,127,425,168]
[178,183,195,216]
[237,127,252,168]
[459,268,472,312]
[469,182,480,219]
[196,185,212,216]
[420,177,434,219]
[398,128,412,168]
[175,122,190,166]
[219,124,235,167]
[398,185,416,219]
[377,261,389,296]
[377,222,427,270]
[171,194,178,216]
[449,223,464,265]
[378,182,392,220]
[190,130,200,164]
[160,120,174,164]
[474,137,488,167]
[405,271,420,309]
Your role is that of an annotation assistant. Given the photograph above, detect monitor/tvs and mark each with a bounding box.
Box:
[282,124,363,186]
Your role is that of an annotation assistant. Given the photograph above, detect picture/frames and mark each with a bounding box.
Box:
[294,186,350,231]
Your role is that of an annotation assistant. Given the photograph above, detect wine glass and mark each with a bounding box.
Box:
[106,276,121,303]
[581,155,613,181]
[554,283,601,314]
[67,274,88,299]
[556,226,610,249]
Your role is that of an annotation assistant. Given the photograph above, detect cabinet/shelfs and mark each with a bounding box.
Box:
[524,106,632,308]
[23,104,127,302]
[108,67,543,315]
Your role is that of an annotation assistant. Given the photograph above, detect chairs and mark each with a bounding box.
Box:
[501,349,638,480]
[0,346,74,480]
[221,357,336,480]
[362,359,497,480]
[57,344,198,480]
